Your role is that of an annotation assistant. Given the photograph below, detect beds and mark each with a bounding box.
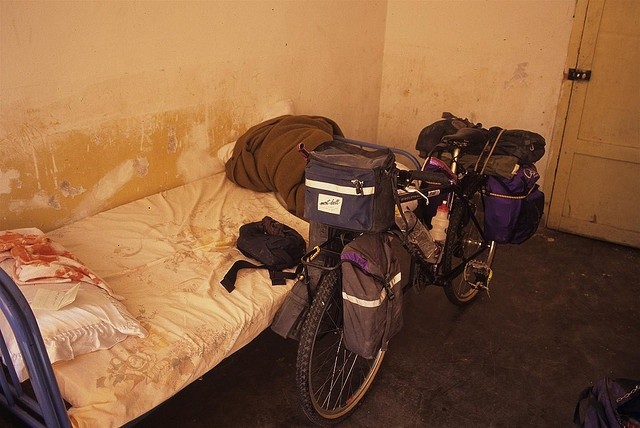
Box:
[0,133,423,428]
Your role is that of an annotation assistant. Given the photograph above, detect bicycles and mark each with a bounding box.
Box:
[297,126,496,427]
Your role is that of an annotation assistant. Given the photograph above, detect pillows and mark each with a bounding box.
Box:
[0,229,149,382]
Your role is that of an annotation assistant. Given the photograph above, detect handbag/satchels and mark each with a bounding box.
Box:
[303,140,395,231]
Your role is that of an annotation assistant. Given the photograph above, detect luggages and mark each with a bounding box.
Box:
[575,375,640,427]
